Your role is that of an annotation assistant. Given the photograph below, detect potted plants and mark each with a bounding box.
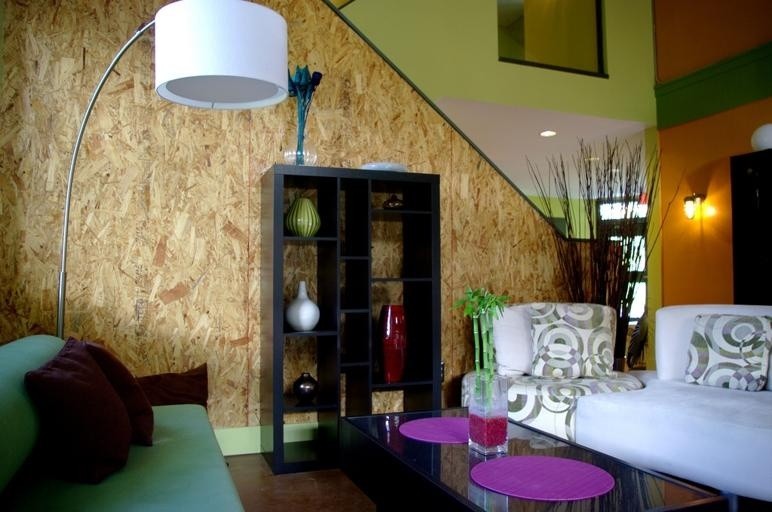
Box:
[449,286,510,452]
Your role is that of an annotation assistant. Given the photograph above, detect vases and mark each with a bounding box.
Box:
[283,130,317,165]
[286,197,320,237]
[293,373,319,406]
[285,280,320,331]
[375,306,406,384]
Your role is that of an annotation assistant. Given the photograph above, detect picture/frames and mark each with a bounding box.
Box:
[496,0,610,79]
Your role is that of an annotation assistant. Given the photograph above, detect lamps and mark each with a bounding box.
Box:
[59,1,289,342]
[751,121,771,150]
[684,191,706,220]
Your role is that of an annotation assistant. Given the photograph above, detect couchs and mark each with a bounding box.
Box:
[0,335,246,512]
[576,304,771,502]
[461,300,642,444]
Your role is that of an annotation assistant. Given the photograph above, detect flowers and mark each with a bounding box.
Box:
[289,65,323,164]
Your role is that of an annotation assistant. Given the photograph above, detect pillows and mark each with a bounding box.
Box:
[133,353,208,408]
[86,342,156,447]
[685,314,769,392]
[24,337,130,485]
[490,309,533,378]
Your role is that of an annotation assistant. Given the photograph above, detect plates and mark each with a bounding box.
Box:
[399,417,470,444]
[470,455,615,501]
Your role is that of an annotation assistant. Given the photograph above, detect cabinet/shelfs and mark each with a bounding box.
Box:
[262,165,441,477]
